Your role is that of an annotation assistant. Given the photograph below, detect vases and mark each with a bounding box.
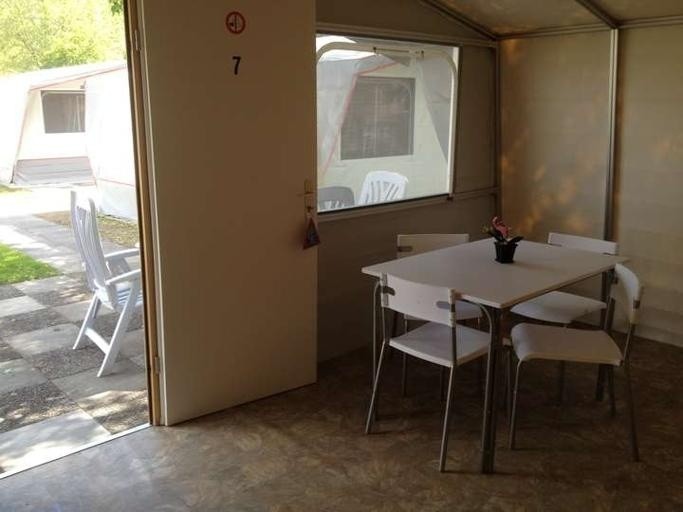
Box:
[493,241,517,264]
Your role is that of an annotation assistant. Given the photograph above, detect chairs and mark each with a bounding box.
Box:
[507,262,645,463]
[317,185,355,212]
[68,189,145,379]
[504,231,619,403]
[363,271,511,472]
[397,232,483,402]
[357,170,409,206]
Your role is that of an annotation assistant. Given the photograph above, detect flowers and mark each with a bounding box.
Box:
[481,216,523,244]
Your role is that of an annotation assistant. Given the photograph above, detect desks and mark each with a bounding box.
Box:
[360,234,631,478]
